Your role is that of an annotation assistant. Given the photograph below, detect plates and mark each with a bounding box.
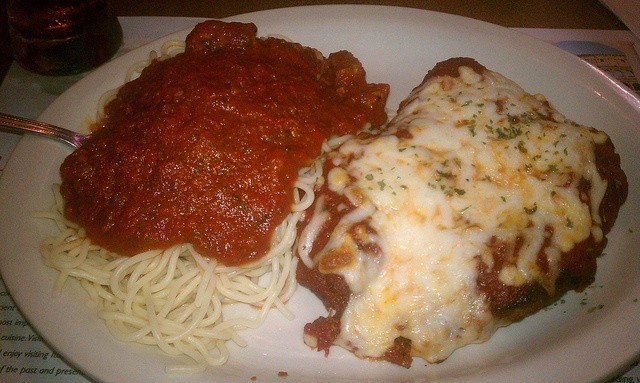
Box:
[0,4,639,382]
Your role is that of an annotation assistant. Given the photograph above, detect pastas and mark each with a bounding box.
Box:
[24,39,370,373]
